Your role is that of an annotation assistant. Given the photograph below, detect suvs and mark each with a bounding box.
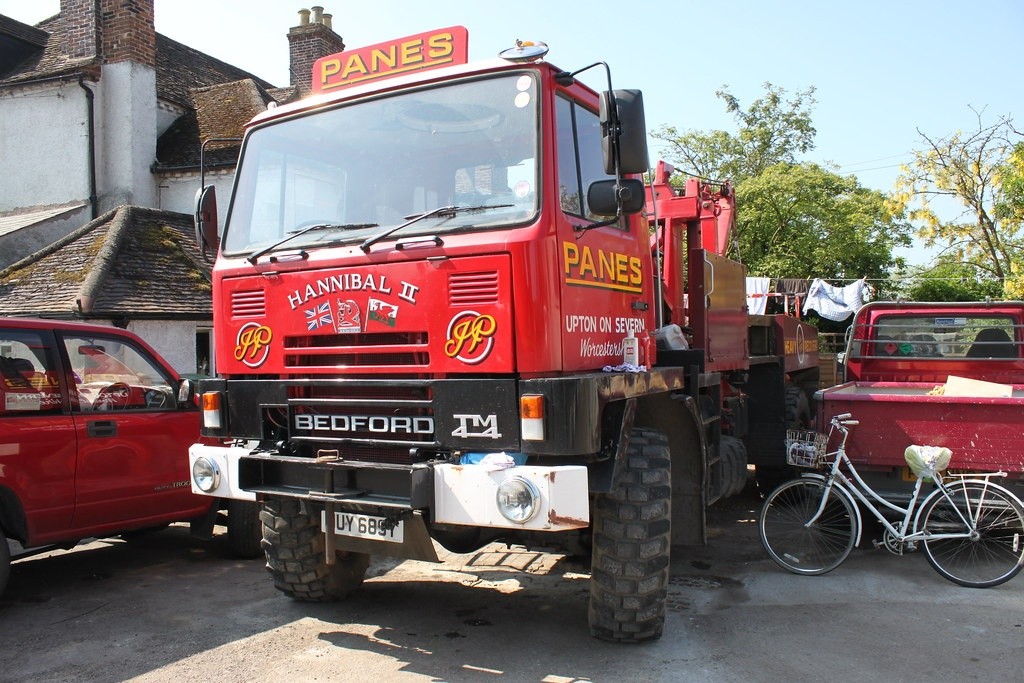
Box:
[0,316,260,601]
[187,24,822,643]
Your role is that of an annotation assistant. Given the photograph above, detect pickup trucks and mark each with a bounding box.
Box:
[812,299,1024,547]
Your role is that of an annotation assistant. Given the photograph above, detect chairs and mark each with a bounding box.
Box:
[966,329,1017,360]
[876,334,905,356]
[909,335,944,358]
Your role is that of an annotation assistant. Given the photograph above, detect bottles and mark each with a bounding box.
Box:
[640,329,651,370]
[623,329,638,367]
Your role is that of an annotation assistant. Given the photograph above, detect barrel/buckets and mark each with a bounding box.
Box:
[656,324,689,350]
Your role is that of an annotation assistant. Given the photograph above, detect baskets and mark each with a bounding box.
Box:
[785,428,830,468]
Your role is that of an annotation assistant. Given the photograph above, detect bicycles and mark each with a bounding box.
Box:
[758,412,1024,589]
